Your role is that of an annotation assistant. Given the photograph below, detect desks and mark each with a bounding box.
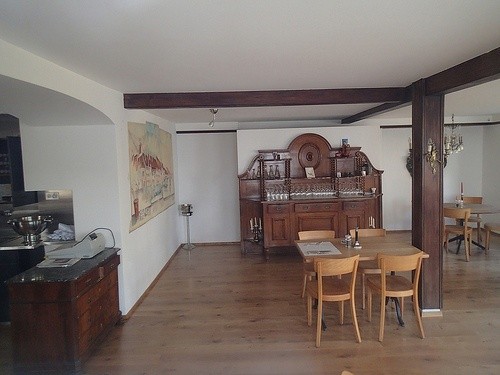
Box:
[440,202,500,255]
[294,237,430,309]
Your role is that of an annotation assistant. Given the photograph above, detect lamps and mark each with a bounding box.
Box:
[444,114,464,155]
[208,108,219,127]
[405,137,414,178]
[424,134,451,175]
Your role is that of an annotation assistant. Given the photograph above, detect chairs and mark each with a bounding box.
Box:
[484,223,500,255]
[350,229,387,310]
[443,208,473,263]
[298,230,342,299]
[366,250,424,342]
[306,254,362,348]
[461,197,482,244]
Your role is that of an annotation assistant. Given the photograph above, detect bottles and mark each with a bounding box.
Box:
[455,196,461,208]
[345,235,353,247]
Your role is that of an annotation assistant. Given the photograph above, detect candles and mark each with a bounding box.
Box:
[373,218,375,228]
[371,216,372,225]
[259,217,262,229]
[369,217,370,226]
[254,217,257,227]
[355,224,358,231]
[250,218,254,230]
[459,181,465,208]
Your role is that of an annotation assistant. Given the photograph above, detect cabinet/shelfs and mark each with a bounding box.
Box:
[238,133,384,263]
[4,247,122,375]
